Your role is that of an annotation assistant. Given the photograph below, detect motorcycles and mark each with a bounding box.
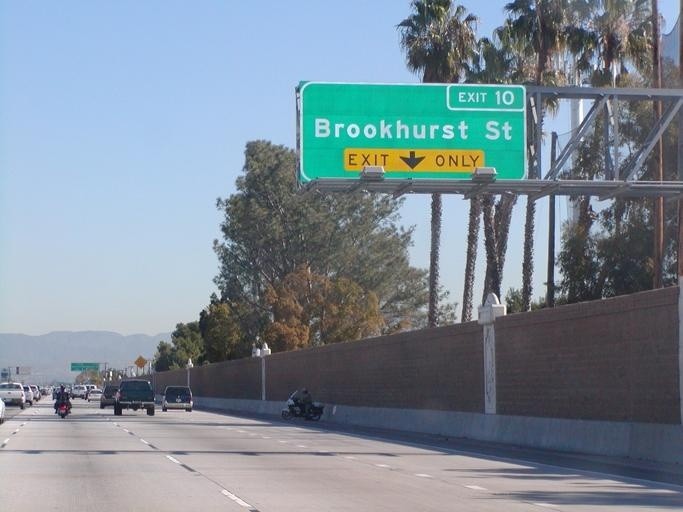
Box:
[281,390,325,422]
[54,392,72,419]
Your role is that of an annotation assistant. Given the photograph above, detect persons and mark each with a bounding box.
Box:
[296,386,313,421]
[52,385,72,415]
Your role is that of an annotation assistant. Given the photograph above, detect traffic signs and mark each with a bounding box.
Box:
[299,79,527,180]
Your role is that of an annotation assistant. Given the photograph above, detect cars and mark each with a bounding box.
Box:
[0,379,50,424]
[160,386,193,412]
[51,379,155,416]
